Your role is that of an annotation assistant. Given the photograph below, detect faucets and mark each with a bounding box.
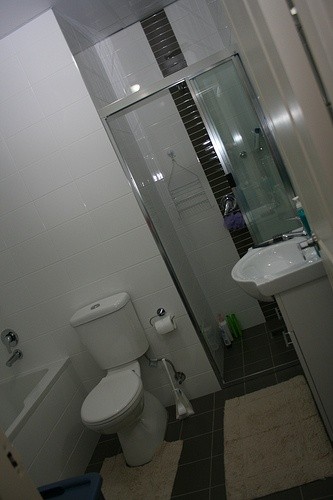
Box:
[6,350,23,367]
[282,216,311,240]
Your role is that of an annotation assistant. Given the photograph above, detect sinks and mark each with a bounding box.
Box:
[231,228,327,298]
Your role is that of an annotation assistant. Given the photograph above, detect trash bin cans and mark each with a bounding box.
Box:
[34,472,105,500]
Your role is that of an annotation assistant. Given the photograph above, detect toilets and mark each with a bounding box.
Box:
[70,292,169,467]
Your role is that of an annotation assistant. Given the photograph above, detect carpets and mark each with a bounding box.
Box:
[222,370,333,499]
[97,438,184,500]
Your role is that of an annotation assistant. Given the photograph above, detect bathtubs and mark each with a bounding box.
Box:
[0,356,103,488]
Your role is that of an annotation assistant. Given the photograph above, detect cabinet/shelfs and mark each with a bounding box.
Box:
[273,275,332,445]
